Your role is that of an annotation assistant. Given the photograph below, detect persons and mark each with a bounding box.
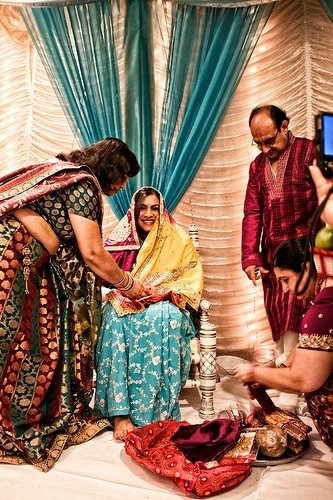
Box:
[0,137,157,472]
[92,186,204,442]
[234,104,333,451]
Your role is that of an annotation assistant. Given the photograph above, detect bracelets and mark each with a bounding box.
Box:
[113,270,135,292]
[248,365,256,382]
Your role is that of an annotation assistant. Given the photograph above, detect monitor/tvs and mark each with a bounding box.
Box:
[316,113,333,157]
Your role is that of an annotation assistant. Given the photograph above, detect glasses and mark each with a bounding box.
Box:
[252,122,284,148]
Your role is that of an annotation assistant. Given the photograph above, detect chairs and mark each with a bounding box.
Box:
[101,225,221,419]
